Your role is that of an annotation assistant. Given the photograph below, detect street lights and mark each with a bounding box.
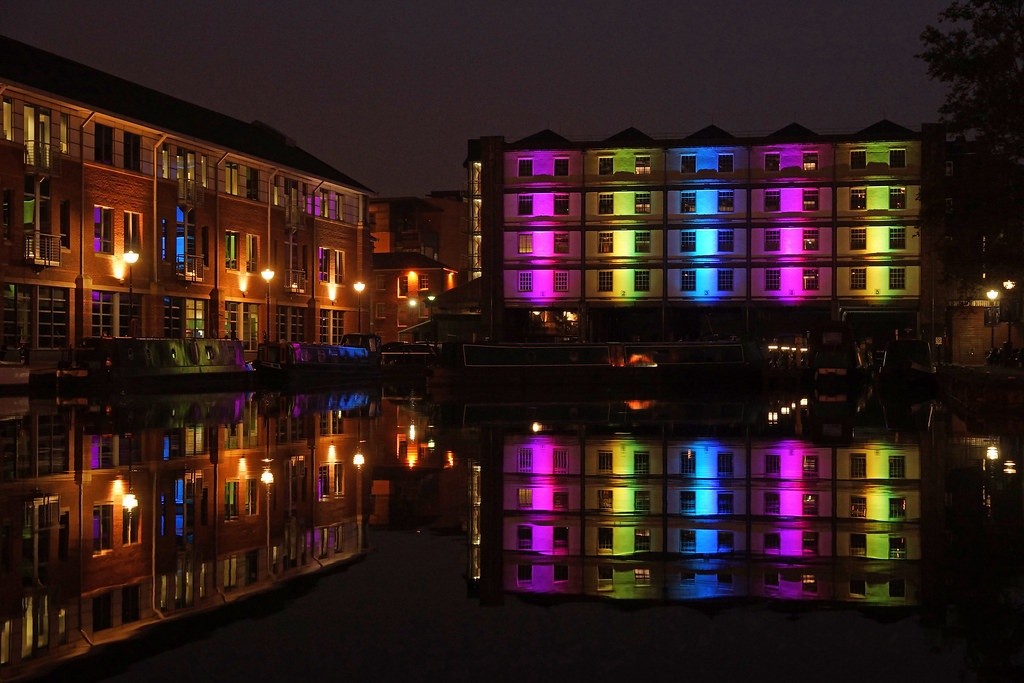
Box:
[988,289,1002,363]
[1002,277,1017,364]
[987,443,998,516]
